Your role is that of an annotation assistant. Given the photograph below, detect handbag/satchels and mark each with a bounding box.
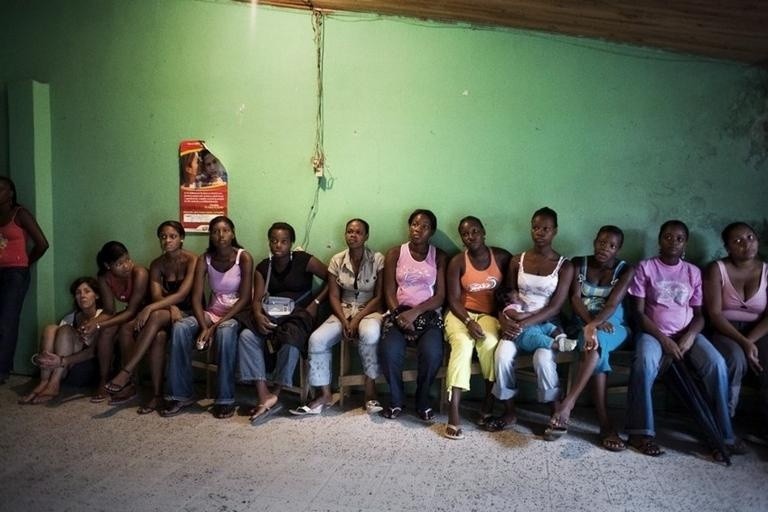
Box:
[261,296,295,319]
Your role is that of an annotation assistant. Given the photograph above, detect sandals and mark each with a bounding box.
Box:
[108,385,138,405]
[710,446,730,463]
[215,405,235,418]
[727,436,751,455]
[102,368,132,394]
[628,435,665,456]
[161,401,195,417]
[90,390,107,403]
[136,394,161,414]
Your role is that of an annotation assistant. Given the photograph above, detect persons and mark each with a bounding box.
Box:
[103,220,200,416]
[438,215,514,441]
[16,276,104,405]
[90,240,150,406]
[236,220,330,425]
[704,221,768,464]
[494,285,578,352]
[379,209,448,422]
[157,216,254,419]
[180,152,202,188]
[624,219,751,458]
[0,175,51,387]
[288,218,385,417]
[544,224,636,451]
[484,206,575,443]
[196,149,227,187]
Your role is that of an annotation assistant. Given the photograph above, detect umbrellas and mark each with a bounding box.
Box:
[666,356,734,467]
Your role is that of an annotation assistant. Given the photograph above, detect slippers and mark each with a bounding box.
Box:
[416,408,436,420]
[545,414,568,435]
[602,437,626,451]
[289,403,323,415]
[444,422,464,439]
[386,407,403,419]
[484,416,516,431]
[17,391,38,405]
[251,394,283,425]
[365,400,384,415]
[30,393,60,405]
[476,408,493,425]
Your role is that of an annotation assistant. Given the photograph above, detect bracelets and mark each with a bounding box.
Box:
[463,316,475,328]
[313,299,321,307]
[95,322,102,331]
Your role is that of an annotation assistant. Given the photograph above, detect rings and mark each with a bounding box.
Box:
[399,319,402,324]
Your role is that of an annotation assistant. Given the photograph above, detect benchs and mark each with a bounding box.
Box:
[339,336,579,413]
[164,338,312,407]
[606,354,768,409]
